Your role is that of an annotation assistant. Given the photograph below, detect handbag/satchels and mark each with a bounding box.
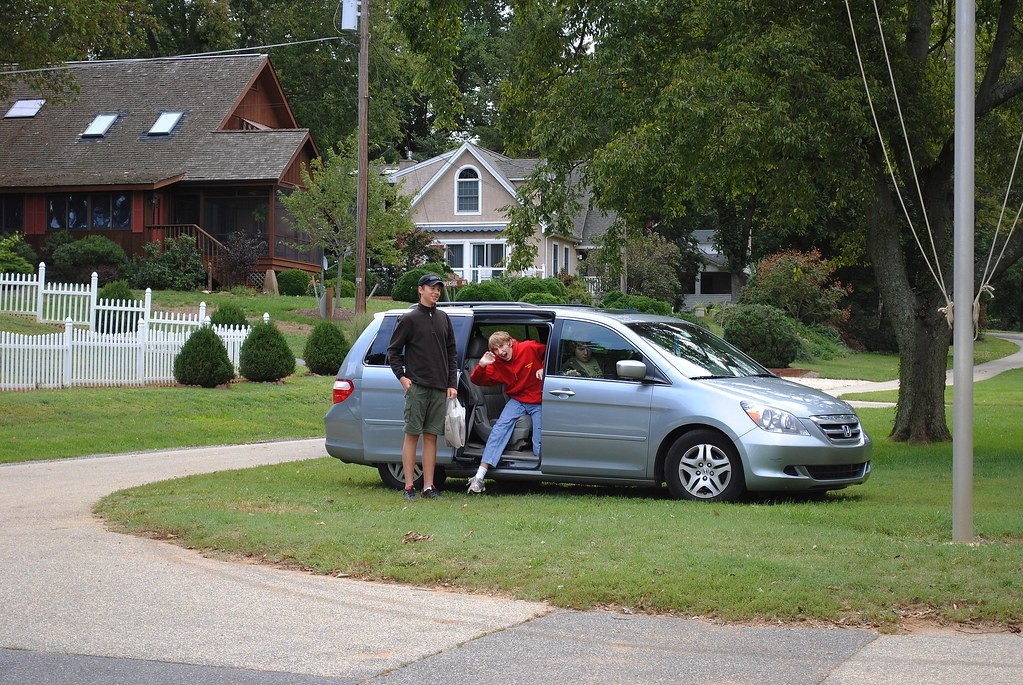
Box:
[444,397,466,449]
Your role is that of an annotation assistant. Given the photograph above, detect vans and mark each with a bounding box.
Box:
[323,297,876,505]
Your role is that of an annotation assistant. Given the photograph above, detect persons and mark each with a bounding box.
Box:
[559,338,618,380]
[388,275,458,501]
[466,331,548,495]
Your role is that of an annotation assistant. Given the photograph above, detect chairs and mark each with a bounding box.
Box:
[465,337,533,450]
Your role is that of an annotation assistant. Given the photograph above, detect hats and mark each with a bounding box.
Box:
[419,274,445,288]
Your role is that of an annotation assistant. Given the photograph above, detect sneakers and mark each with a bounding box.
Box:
[402,485,418,502]
[465,476,486,494]
[420,484,441,501]
